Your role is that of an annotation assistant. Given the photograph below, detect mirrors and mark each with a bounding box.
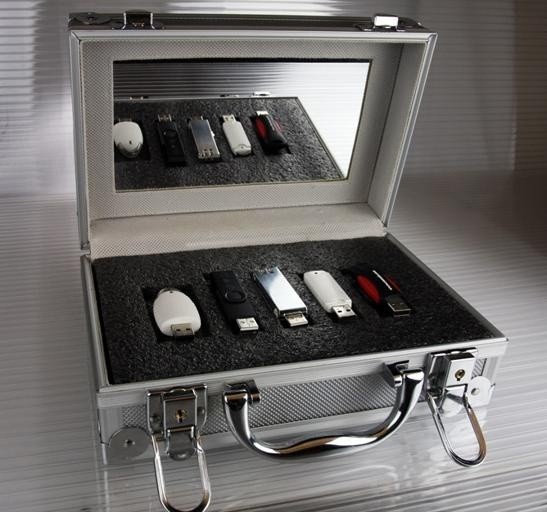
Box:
[114,61,373,193]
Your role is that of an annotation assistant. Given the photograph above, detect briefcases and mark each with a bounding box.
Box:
[64,7,511,512]
